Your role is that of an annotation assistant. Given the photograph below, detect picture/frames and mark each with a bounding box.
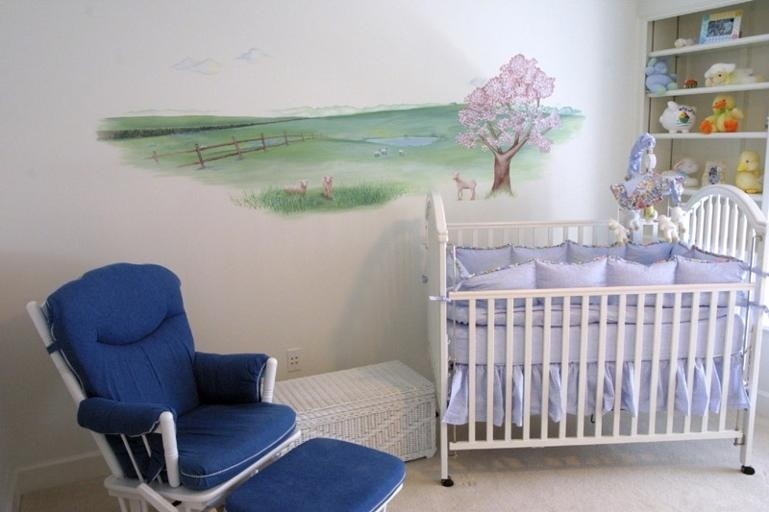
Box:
[697,8,745,42]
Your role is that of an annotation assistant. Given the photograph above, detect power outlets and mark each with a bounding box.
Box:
[287,347,303,372]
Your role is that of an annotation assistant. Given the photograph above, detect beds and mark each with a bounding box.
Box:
[423,181,767,489]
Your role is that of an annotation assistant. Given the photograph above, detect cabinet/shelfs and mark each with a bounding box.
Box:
[641,0,769,216]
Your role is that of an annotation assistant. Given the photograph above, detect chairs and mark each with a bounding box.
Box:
[25,262,302,511]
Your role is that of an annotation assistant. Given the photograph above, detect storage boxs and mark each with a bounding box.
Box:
[251,357,438,477]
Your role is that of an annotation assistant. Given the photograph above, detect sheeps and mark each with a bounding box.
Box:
[286,179,309,194]
[322,176,334,197]
[452,171,477,201]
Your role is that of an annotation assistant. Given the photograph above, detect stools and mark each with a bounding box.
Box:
[226,434,408,511]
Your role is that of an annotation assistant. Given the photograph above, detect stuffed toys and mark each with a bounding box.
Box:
[736,149,764,194]
[659,101,697,133]
[607,206,687,243]
[673,157,700,187]
[643,58,678,96]
[700,94,744,134]
[704,62,764,87]
[609,131,685,209]
[701,160,728,188]
[683,80,697,89]
